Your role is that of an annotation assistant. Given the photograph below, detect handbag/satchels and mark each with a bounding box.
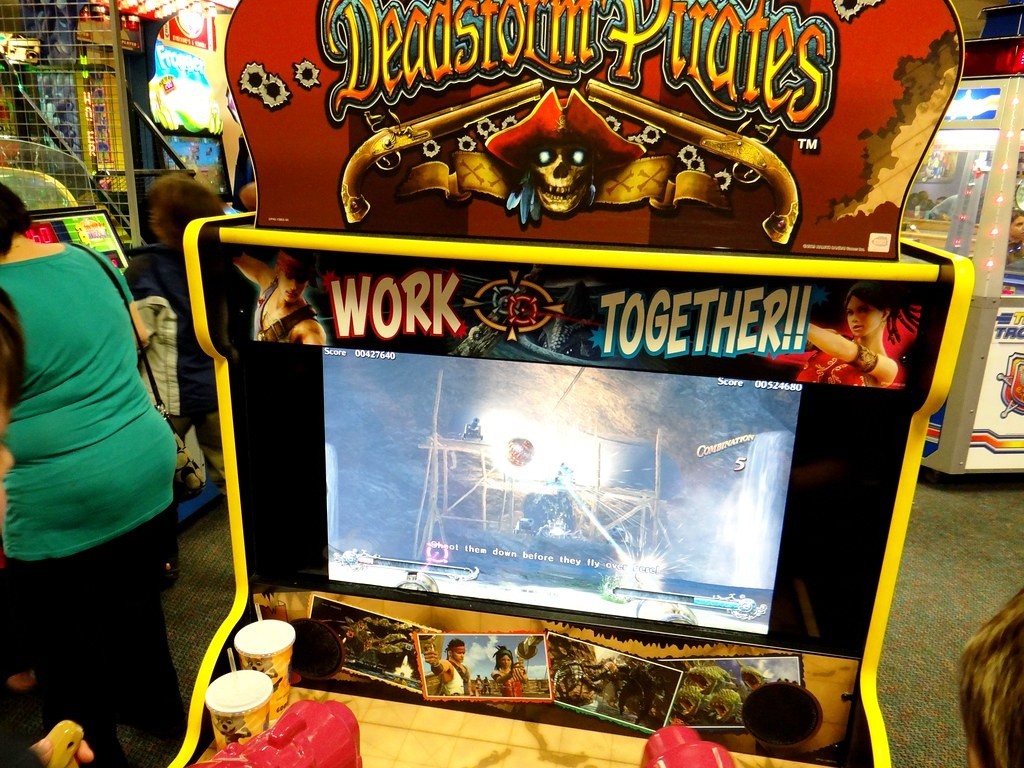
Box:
[162,413,207,500]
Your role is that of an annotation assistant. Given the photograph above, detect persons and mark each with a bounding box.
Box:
[0,181,230,768]
[902,189,1024,264]
[124,173,225,486]
[959,589,1023,768]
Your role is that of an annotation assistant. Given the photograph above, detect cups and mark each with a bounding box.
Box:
[234,619,296,721]
[205,670,274,753]
